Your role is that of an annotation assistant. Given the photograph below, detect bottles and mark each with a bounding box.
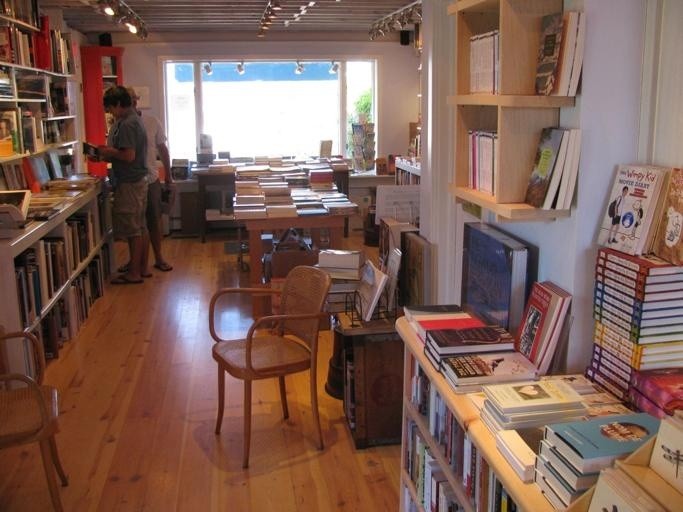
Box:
[126,88,140,100]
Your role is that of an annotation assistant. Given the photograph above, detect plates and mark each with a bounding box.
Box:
[161,170,395,243]
[393,311,683,512]
[336,303,464,451]
[444,0,577,222]
[78,44,126,177]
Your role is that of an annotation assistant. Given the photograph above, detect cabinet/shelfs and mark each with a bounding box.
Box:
[161,170,395,243]
[444,0,577,222]
[1,15,119,392]
[78,44,126,177]
[393,311,683,512]
[336,303,464,451]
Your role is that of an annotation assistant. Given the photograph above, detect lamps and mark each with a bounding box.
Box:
[236,61,245,76]
[294,61,306,76]
[329,61,338,75]
[0,330,69,511]
[255,1,282,41]
[368,3,422,43]
[97,0,141,35]
[207,264,333,470]
[203,62,213,76]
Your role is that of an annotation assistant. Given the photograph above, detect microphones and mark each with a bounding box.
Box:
[204,208,250,273]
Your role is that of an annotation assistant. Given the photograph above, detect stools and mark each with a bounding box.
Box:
[204,208,250,273]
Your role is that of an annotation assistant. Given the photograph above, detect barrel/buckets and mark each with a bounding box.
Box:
[111,260,172,284]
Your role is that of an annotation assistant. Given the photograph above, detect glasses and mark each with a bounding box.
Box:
[255,1,282,41]
[368,3,422,43]
[294,61,306,76]
[203,62,213,76]
[236,61,245,76]
[329,61,338,75]
[97,0,141,35]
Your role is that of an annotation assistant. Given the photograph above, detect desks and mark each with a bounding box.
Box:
[232,209,355,318]
[196,172,351,244]
[392,161,421,186]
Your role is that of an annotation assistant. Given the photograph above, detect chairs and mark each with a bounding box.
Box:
[0,330,69,511]
[608,238,618,244]
[207,264,333,470]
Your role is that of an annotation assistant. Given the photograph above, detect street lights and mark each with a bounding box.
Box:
[608,195,622,217]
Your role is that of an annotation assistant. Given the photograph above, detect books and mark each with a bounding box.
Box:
[2,1,81,190]
[197,152,230,165]
[393,125,421,185]
[536,13,587,97]
[320,141,332,158]
[234,157,350,221]
[469,129,497,195]
[468,30,499,94]
[346,362,356,427]
[402,223,683,512]
[597,166,681,265]
[525,127,582,210]
[172,159,189,180]
[0,174,119,361]
[311,217,433,322]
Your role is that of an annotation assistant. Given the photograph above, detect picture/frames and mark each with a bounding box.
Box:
[196,172,351,244]
[232,209,355,318]
[392,161,421,186]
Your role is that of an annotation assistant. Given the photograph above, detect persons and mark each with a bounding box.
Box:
[97,85,152,284]
[118,88,173,273]
